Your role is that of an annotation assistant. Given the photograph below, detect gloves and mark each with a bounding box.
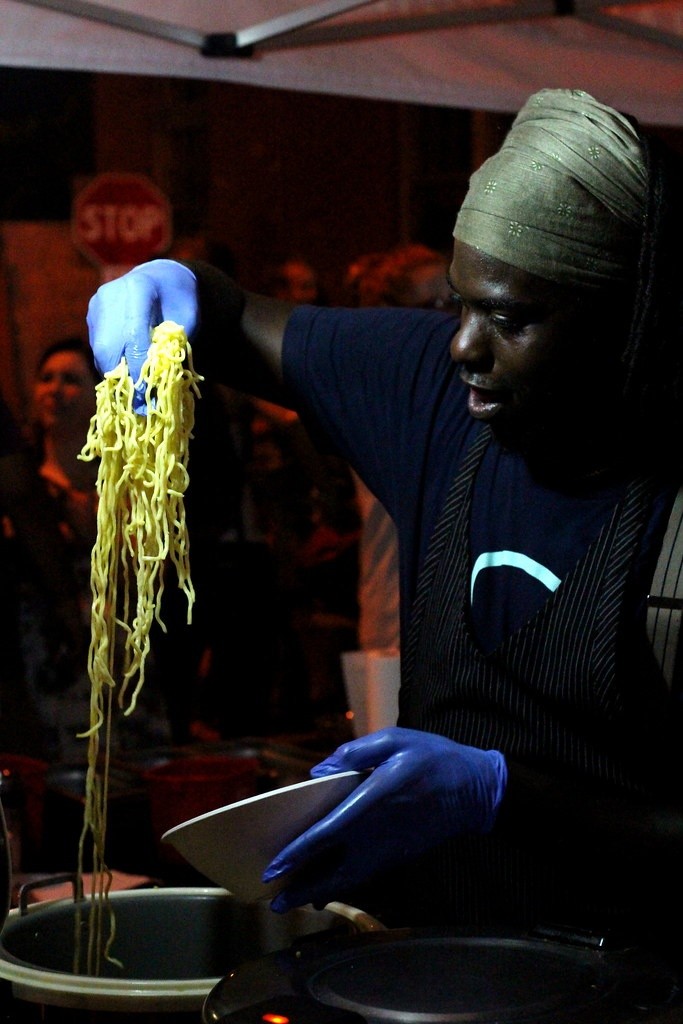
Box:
[84,256,201,421]
[264,726,509,912]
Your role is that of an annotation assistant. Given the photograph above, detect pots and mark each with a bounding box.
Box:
[203,922,683,1023]
[0,873,388,1024]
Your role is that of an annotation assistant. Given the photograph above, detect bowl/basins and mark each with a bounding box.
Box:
[162,766,377,903]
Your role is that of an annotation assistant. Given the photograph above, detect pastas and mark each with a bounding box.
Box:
[76,321,202,984]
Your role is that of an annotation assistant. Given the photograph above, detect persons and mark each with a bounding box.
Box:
[0,240,462,765]
[88,89,682,1024]
